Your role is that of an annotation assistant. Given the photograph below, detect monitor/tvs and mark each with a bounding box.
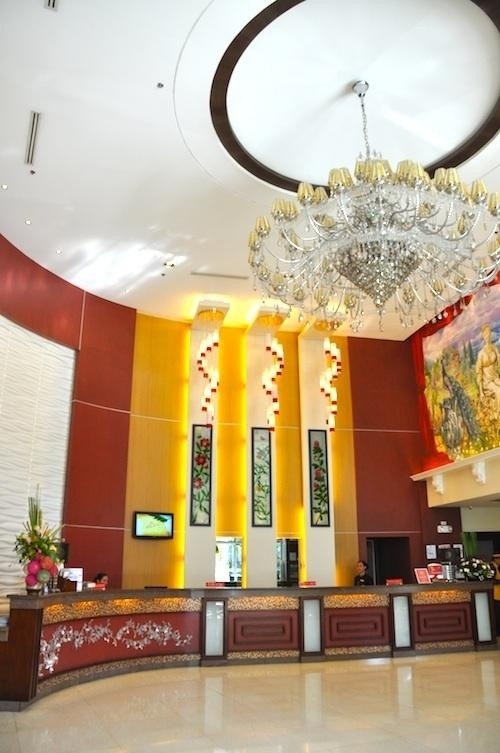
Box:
[133,511,173,539]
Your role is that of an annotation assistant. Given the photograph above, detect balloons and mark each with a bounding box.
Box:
[26,555,58,586]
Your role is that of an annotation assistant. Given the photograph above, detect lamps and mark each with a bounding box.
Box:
[246,76,500,344]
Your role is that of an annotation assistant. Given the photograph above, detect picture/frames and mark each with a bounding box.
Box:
[307,427,332,527]
[190,423,214,527]
[251,426,274,529]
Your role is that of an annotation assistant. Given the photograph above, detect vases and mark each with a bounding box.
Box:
[23,581,43,596]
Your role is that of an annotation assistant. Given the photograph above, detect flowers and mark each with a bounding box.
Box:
[11,483,67,588]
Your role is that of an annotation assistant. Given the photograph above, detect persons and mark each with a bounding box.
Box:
[474,323,499,402]
[354,560,373,586]
[93,573,110,586]
